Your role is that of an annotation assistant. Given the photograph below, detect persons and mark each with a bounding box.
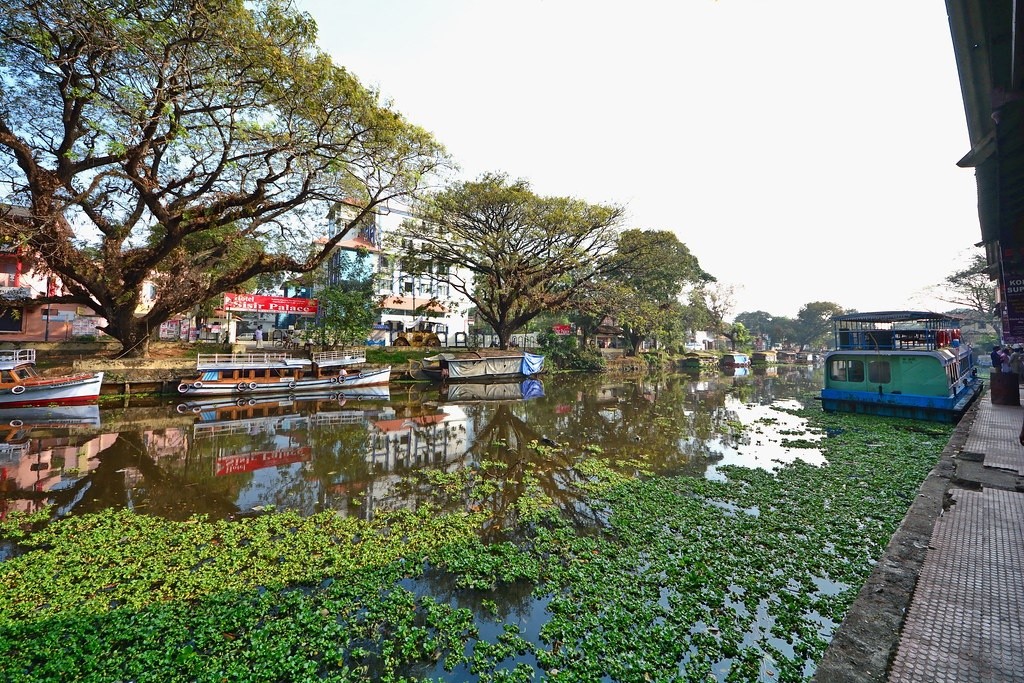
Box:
[991,343,1024,384]
[283,330,313,351]
[220,331,229,350]
[253,326,263,348]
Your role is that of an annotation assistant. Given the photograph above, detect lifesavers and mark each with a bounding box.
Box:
[358,396,364,401]
[358,373,364,379]
[249,399,256,406]
[194,381,203,389]
[9,420,23,427]
[289,381,296,388]
[249,382,258,389]
[238,381,248,391]
[289,395,296,402]
[192,407,202,414]
[338,392,345,401]
[338,375,346,383]
[12,385,25,395]
[177,403,188,414]
[237,399,247,408]
[177,382,190,394]
[330,377,337,383]
[329,393,337,401]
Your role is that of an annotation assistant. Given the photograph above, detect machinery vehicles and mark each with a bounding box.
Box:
[393,319,447,348]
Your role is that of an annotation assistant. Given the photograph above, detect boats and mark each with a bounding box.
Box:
[0,402,102,429]
[684,352,720,368]
[422,347,546,380]
[437,377,547,404]
[820,310,985,424]
[0,349,105,404]
[722,353,752,366]
[176,399,395,442]
[178,347,394,397]
